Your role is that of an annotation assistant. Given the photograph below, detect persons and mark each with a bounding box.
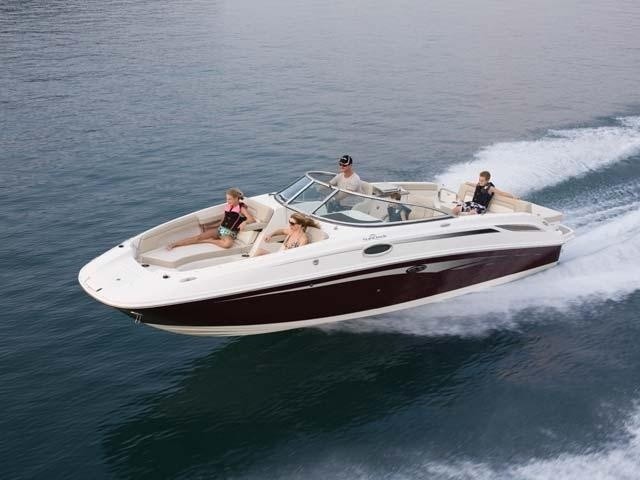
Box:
[451,171,520,216]
[318,155,365,213]
[382,193,406,222]
[255,213,320,256]
[167,187,256,251]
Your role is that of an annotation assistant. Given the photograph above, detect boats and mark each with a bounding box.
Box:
[74,170,575,343]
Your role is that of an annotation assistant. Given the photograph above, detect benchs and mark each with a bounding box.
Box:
[434,183,531,218]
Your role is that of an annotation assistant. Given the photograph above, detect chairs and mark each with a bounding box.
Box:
[266,224,330,252]
[137,198,275,269]
[343,181,374,215]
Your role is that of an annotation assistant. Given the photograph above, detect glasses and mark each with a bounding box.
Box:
[339,164,347,167]
[289,219,299,225]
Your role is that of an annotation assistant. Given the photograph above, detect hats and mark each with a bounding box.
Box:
[338,154,352,166]
